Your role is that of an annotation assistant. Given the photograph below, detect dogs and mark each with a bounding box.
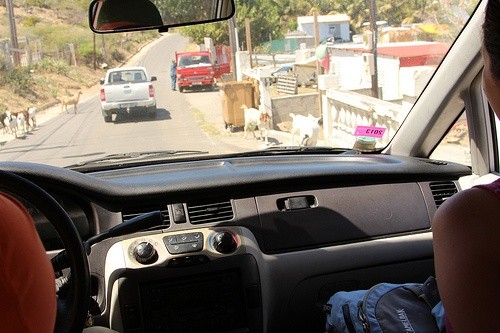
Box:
[289,112,323,146]
[241,103,271,145]
[61,91,84,114]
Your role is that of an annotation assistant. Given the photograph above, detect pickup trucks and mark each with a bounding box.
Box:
[98,67,158,123]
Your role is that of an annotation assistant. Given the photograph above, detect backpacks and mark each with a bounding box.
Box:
[320,275,446,333]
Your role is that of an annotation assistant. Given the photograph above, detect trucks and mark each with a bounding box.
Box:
[174,49,231,92]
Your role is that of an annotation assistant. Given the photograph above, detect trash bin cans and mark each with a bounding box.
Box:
[219,79,254,132]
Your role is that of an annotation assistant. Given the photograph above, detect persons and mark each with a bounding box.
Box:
[0,192,118,333]
[431,0,500,333]
[170,60,177,90]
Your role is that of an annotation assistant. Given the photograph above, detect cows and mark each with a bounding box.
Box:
[0,106,36,137]
